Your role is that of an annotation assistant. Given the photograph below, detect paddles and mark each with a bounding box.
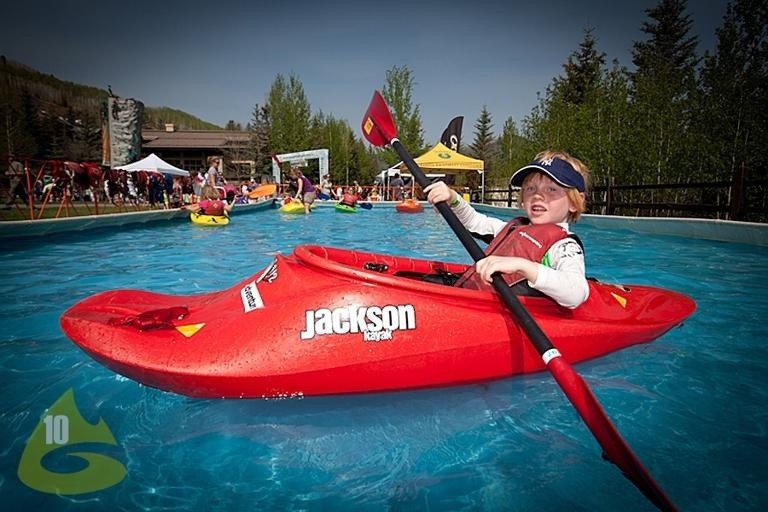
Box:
[271,152,296,192]
[318,193,373,210]
[362,89,673,511]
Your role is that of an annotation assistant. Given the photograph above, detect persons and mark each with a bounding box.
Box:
[423,150,590,309]
[2,154,29,210]
[163,156,404,215]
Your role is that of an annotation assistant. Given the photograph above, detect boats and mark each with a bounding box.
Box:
[59,244,697,400]
[398,200,424,212]
[281,198,317,214]
[334,200,358,213]
[190,202,230,226]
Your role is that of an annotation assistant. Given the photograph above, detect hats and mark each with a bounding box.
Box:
[510,158,585,193]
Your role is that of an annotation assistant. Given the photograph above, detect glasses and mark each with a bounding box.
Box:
[214,161,219,164]
